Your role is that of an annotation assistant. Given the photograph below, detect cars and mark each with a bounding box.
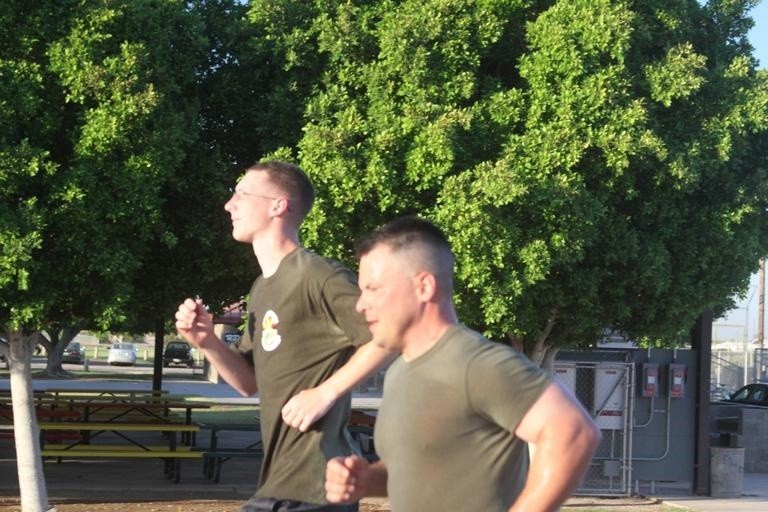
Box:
[106,342,138,367]
[719,383,768,406]
[61,342,86,364]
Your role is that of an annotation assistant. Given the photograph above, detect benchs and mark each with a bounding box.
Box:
[1,387,377,483]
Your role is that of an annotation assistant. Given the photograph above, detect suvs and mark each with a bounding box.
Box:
[163,342,194,369]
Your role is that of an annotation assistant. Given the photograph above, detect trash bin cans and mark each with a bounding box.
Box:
[708,445,744,499]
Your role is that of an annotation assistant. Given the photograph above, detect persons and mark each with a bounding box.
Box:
[174,165,400,512]
[324,219,602,511]
